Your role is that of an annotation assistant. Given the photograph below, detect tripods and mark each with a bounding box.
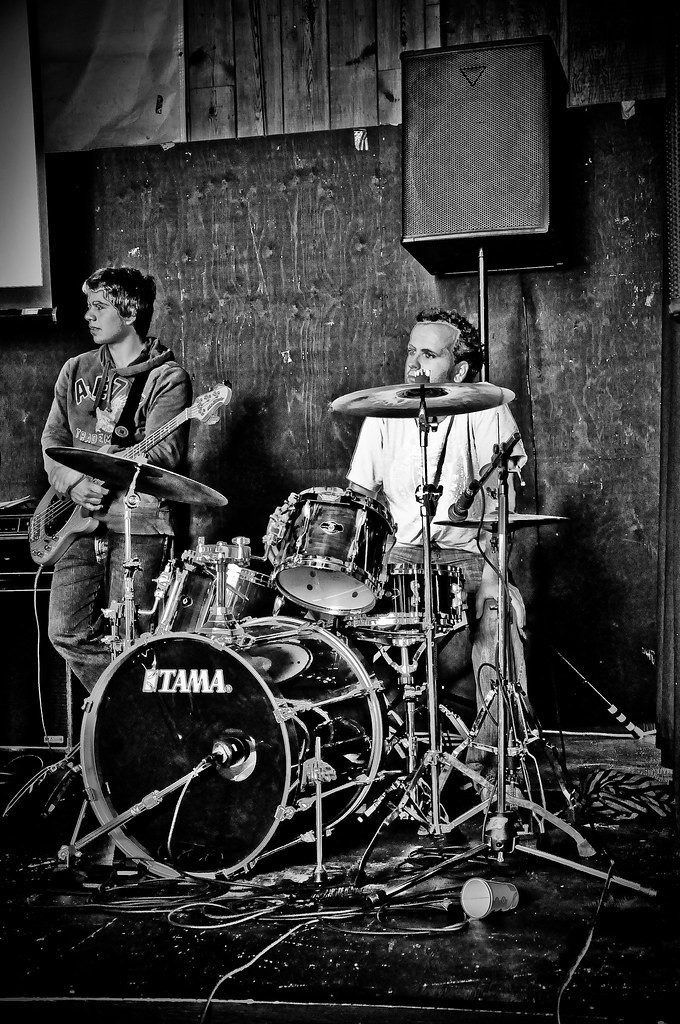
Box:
[39,384,657,912]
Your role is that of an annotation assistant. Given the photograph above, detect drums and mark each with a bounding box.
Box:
[272,487,397,615]
[155,555,272,637]
[346,563,467,630]
[79,617,384,877]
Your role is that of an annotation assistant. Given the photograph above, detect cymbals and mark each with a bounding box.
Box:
[332,382,516,419]
[44,445,228,507]
[434,512,568,532]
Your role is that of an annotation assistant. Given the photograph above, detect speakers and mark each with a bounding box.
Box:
[399,35,571,277]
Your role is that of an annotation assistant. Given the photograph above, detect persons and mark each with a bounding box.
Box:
[38,266,192,696]
[342,308,539,851]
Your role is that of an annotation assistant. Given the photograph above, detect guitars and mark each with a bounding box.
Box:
[29,385,232,565]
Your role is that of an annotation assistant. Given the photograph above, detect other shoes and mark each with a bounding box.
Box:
[471,771,525,806]
